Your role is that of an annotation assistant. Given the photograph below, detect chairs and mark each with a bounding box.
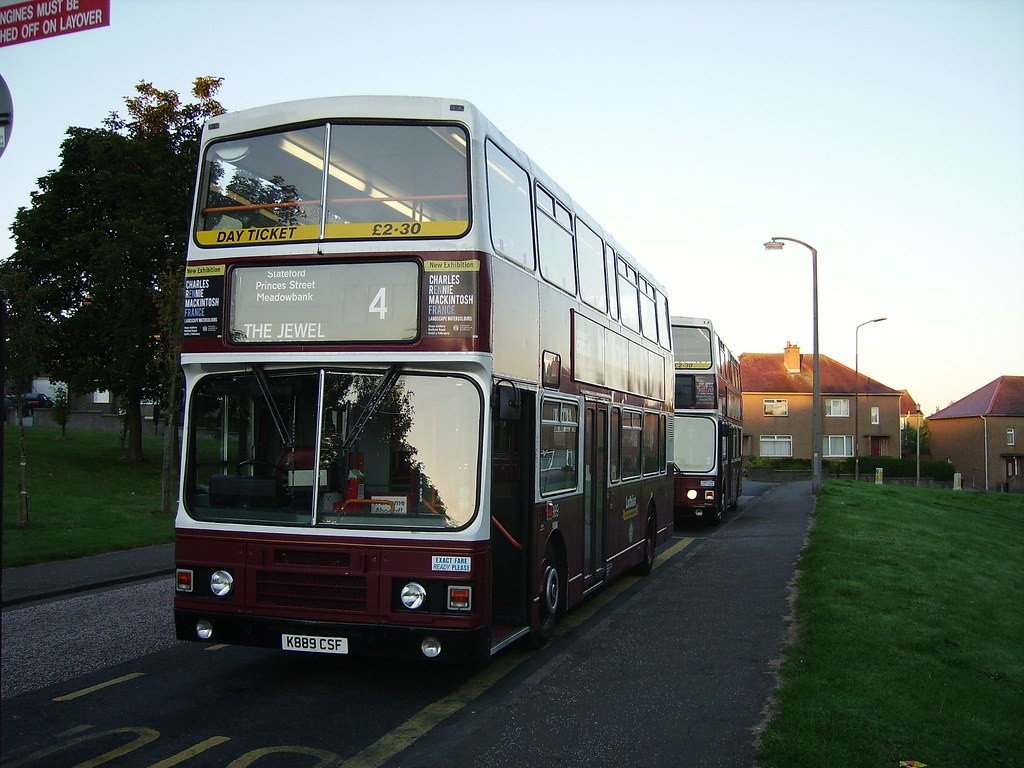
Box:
[208,446,345,511]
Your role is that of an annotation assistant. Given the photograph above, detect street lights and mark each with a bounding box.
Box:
[854,318,888,480]
[763,237,824,493]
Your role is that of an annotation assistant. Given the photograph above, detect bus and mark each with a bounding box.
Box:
[666,318,743,530]
[175,93,675,672]
